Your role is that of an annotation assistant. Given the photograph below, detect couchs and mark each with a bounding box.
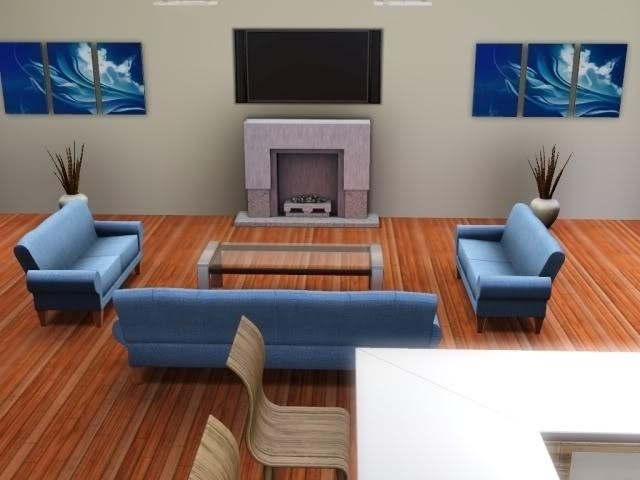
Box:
[112,289,441,370]
[456,203,565,333]
[14,201,144,330]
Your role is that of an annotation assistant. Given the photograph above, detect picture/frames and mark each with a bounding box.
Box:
[471,40,627,119]
[0,41,148,116]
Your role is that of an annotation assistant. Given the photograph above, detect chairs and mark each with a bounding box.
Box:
[227,315,351,479]
[191,414,243,480]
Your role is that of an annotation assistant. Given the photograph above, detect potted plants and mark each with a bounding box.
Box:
[43,140,93,208]
[527,145,575,229]
